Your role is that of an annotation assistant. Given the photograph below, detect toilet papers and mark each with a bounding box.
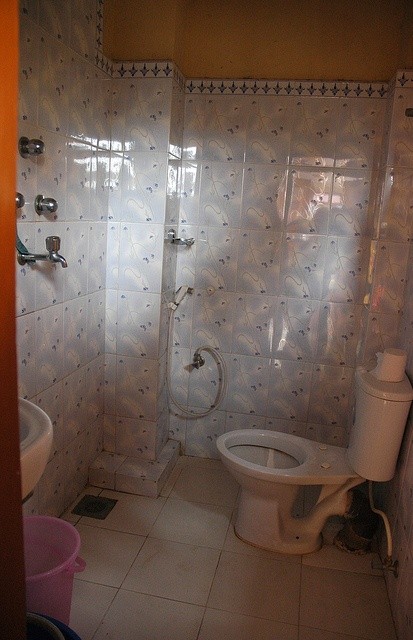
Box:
[370,348,408,382]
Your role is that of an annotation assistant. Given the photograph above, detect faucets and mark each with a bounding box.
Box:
[17,236,69,268]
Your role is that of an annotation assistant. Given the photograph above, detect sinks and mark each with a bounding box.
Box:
[18,396,54,500]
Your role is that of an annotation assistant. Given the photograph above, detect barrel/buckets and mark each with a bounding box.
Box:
[22,515,85,628]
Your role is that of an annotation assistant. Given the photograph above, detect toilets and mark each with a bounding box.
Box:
[216,370,413,554]
